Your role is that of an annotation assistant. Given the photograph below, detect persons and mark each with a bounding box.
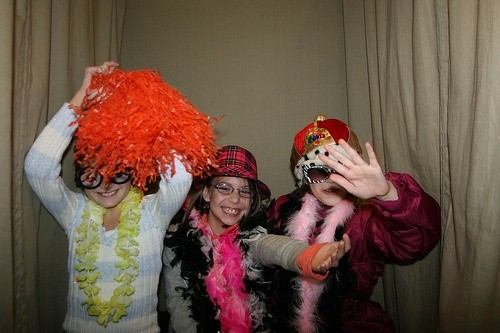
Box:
[265,114,442,333]
[23,61,194,333]
[162,145,352,333]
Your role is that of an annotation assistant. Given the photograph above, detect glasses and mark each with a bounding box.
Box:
[74,165,132,189]
[303,166,331,183]
[210,182,256,198]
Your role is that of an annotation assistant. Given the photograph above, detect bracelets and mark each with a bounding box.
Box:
[375,181,392,198]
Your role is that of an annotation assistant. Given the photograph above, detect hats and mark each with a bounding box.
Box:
[290,113,363,187]
[212,145,271,201]
